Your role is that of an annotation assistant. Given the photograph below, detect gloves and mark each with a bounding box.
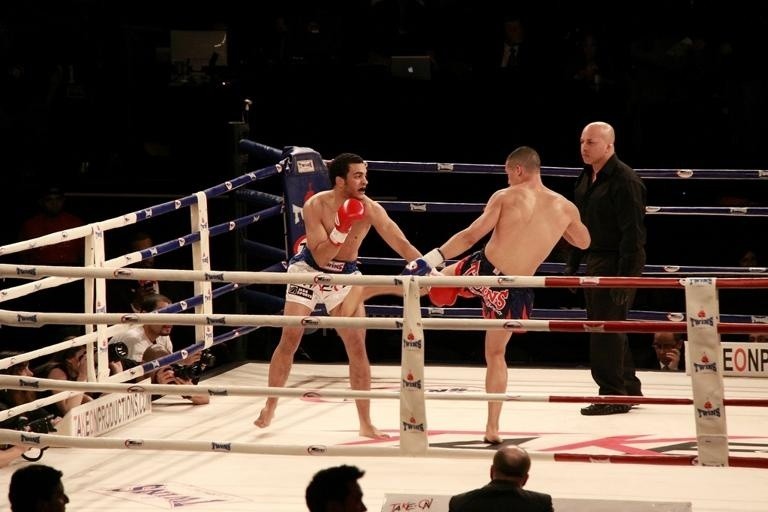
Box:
[399,260,428,279]
[564,271,579,293]
[429,286,457,308]
[328,197,364,246]
[608,287,630,307]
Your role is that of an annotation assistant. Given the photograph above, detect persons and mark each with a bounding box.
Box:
[635,332,685,372]
[574,123,644,416]
[447,445,555,511]
[8,465,67,512]
[252,155,441,439]
[339,144,590,443]
[304,464,368,511]
[1,183,209,448]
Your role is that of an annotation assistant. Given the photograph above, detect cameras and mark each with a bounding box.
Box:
[171,363,201,380]
[27,414,56,450]
[201,348,216,370]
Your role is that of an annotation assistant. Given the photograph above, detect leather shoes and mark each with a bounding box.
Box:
[580,404,630,415]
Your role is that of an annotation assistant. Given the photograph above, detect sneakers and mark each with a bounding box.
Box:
[652,343,677,350]
